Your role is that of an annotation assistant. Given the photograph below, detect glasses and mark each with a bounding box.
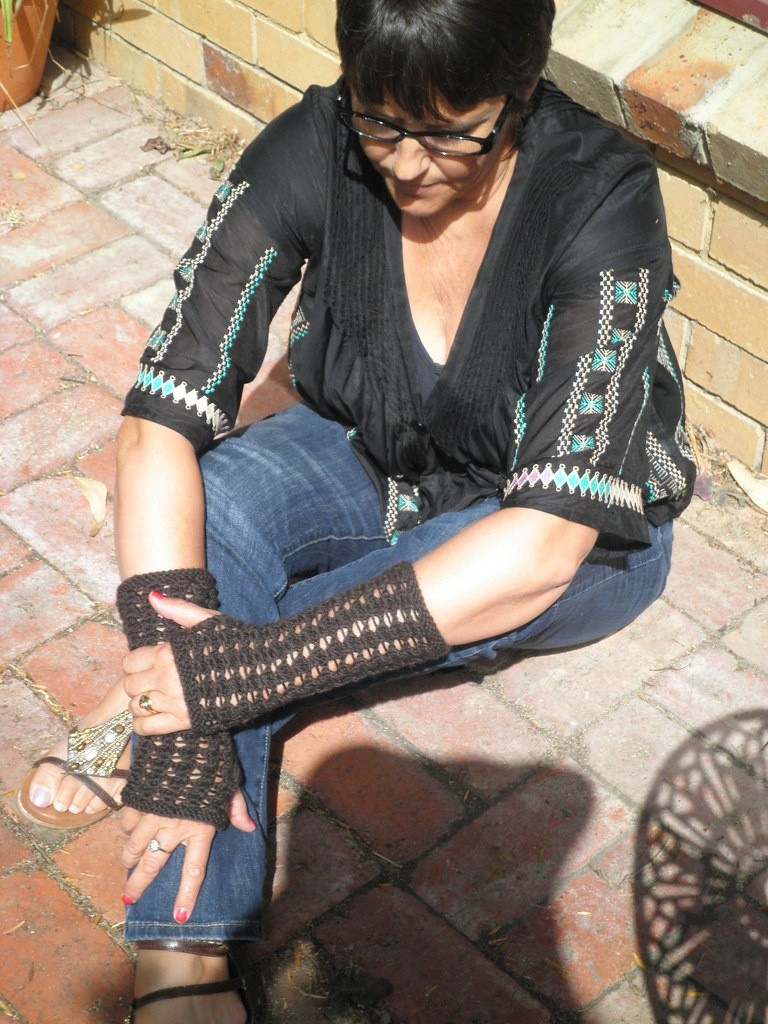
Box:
[336,77,514,157]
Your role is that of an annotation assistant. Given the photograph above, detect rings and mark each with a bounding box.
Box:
[147,839,173,854]
[139,695,157,718]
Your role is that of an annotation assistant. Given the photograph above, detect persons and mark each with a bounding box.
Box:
[18,0,696,1024]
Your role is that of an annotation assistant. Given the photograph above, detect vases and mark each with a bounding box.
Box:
[0,0,57,113]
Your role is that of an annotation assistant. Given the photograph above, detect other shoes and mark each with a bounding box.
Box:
[125,938,252,1024]
[15,709,133,830]
